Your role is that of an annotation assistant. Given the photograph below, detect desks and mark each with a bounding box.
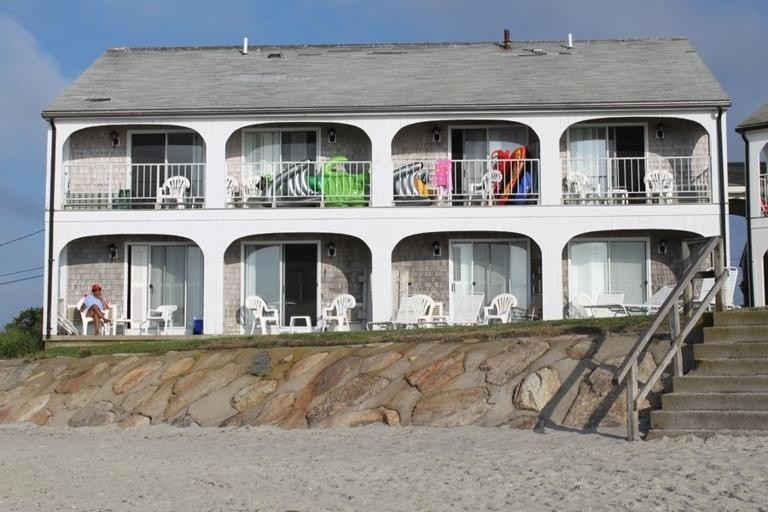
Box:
[288,314,313,334]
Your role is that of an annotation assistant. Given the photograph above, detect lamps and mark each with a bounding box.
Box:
[326,127,337,145]
[432,240,443,257]
[654,121,666,141]
[325,239,336,258]
[105,242,120,262]
[655,236,669,255]
[107,130,123,147]
[432,126,442,143]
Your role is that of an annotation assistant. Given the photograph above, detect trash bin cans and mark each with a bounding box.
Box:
[193,320,203,335]
[119,190,130,209]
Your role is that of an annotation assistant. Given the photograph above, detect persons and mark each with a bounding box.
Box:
[79,284,111,335]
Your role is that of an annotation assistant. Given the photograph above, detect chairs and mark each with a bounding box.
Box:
[75,296,178,336]
[363,283,676,331]
[320,293,356,331]
[564,169,674,207]
[155,177,189,210]
[246,295,280,336]
[466,171,502,207]
[224,173,266,207]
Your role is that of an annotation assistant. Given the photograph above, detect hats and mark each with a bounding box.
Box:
[92,284,101,293]
